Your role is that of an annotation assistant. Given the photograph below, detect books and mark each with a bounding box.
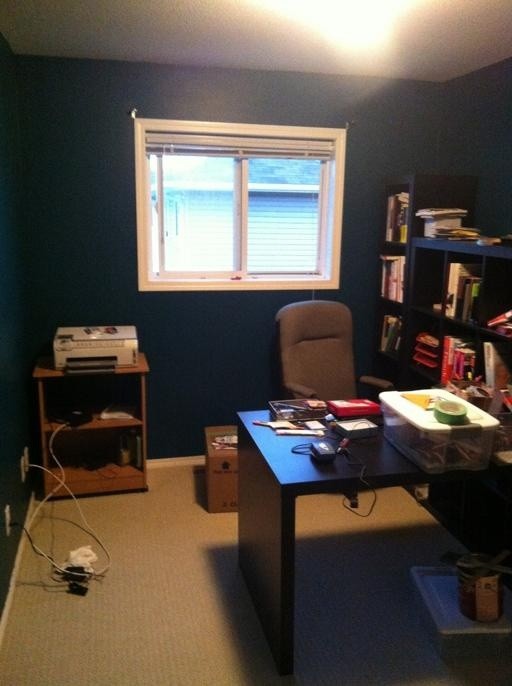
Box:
[327,398,381,419]
[100,399,136,420]
[268,395,329,423]
[377,188,511,419]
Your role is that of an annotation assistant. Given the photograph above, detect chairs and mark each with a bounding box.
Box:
[271,297,396,409]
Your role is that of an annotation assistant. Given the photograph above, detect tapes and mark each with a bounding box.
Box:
[434,400,467,424]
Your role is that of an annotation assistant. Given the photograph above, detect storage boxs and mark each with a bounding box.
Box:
[201,423,241,513]
[407,561,509,660]
[375,385,500,476]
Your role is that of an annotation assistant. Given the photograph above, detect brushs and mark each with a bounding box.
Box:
[440,549,511,575]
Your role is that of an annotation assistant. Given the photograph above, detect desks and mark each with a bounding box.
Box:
[234,408,510,685]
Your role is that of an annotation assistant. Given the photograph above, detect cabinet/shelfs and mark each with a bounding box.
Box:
[373,171,511,456]
[32,353,150,500]
